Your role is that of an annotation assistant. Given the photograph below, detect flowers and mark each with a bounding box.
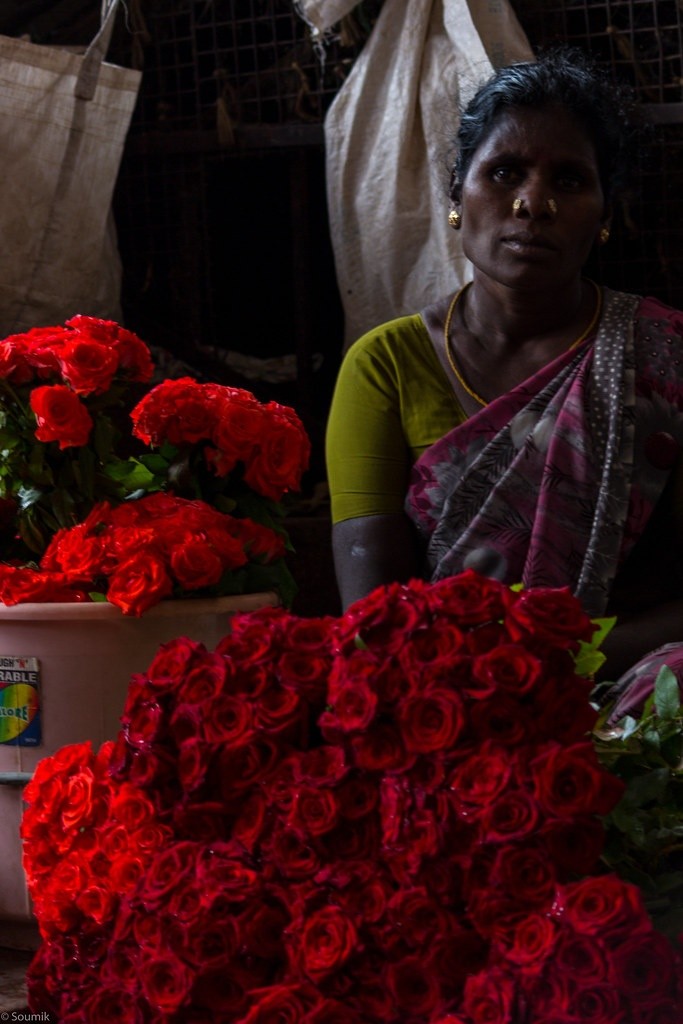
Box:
[0,314,323,618]
[19,572,683,1023]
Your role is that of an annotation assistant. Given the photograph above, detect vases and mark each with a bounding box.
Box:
[0,594,282,952]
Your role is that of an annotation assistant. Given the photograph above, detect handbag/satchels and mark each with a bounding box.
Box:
[0,0,143,341]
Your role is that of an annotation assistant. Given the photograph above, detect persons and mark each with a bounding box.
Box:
[325,65,683,729]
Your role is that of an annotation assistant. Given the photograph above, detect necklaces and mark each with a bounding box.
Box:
[445,276,603,407]
